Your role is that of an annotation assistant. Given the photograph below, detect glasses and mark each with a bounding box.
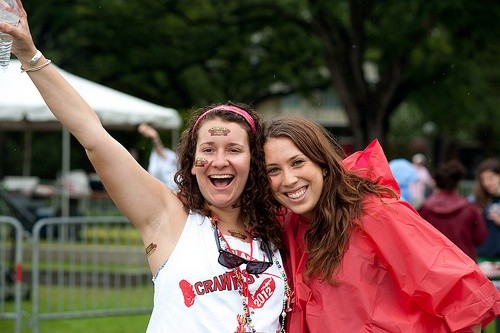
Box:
[214,220,273,274]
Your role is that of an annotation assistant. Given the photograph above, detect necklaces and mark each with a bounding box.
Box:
[209,217,287,333]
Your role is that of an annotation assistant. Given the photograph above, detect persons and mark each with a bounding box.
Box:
[415,159,486,264]
[389,158,421,204]
[55,162,91,241]
[175,116,500,333]
[411,153,435,209]
[137,123,183,196]
[0,0,292,333]
[467,157,499,281]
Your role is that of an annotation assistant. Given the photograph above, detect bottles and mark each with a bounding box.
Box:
[0,0,19,69]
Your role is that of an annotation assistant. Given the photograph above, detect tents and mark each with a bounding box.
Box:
[0,59,182,242]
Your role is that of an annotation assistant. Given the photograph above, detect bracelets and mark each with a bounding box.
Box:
[21,59,51,73]
[21,50,42,70]
